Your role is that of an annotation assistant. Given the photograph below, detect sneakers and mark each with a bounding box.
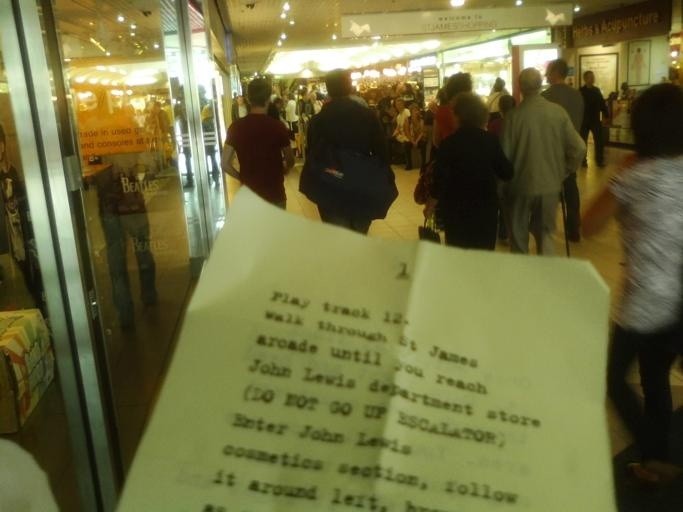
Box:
[627,463,663,485]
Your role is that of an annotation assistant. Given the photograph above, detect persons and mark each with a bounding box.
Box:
[423,92,516,255]
[2,132,48,314]
[497,66,588,255]
[229,73,438,171]
[630,44,646,83]
[539,59,584,241]
[486,78,510,128]
[486,95,516,132]
[218,77,296,216]
[572,82,682,489]
[578,70,609,168]
[416,72,473,212]
[76,83,155,339]
[301,67,398,240]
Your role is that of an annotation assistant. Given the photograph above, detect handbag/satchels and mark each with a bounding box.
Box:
[418,227,439,244]
[300,143,398,219]
[411,148,423,168]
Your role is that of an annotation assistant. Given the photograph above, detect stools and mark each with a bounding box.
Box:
[0,308,54,434]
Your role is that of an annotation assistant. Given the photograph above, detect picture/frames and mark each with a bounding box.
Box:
[626,39,652,87]
